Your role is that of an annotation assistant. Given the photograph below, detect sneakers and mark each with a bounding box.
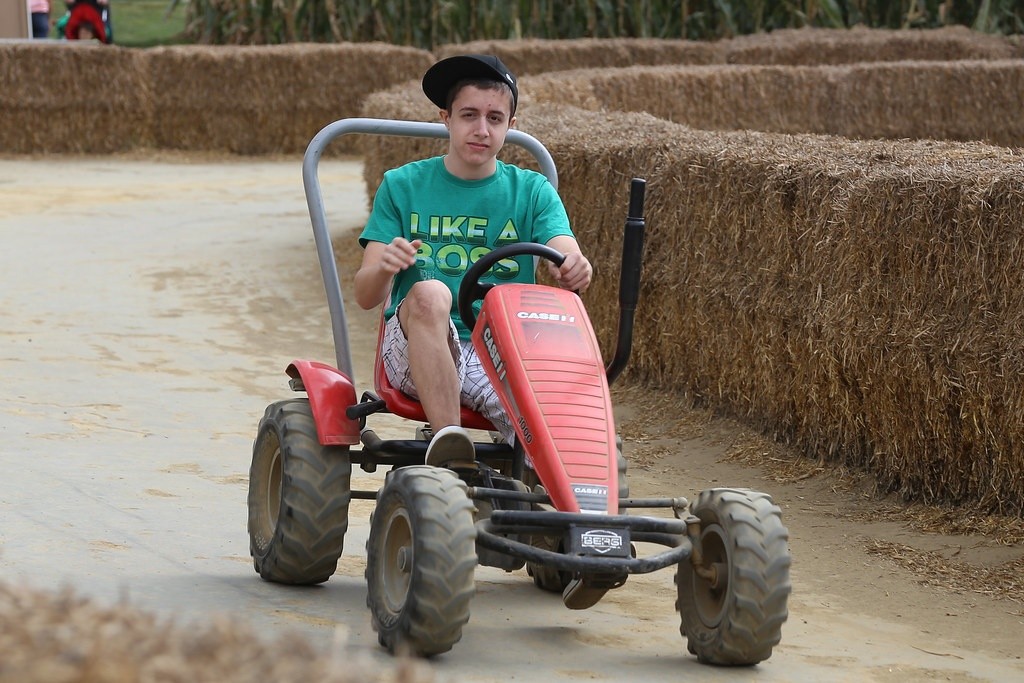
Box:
[425,424,476,468]
[562,542,636,610]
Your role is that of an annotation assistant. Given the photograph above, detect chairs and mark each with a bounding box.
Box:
[374,291,498,432]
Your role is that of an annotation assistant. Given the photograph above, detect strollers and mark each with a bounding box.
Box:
[63,1,114,44]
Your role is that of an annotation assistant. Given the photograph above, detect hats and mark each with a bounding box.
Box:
[422,54,518,109]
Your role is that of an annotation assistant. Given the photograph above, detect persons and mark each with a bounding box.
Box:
[30,0,112,45]
[354,56,637,611]
